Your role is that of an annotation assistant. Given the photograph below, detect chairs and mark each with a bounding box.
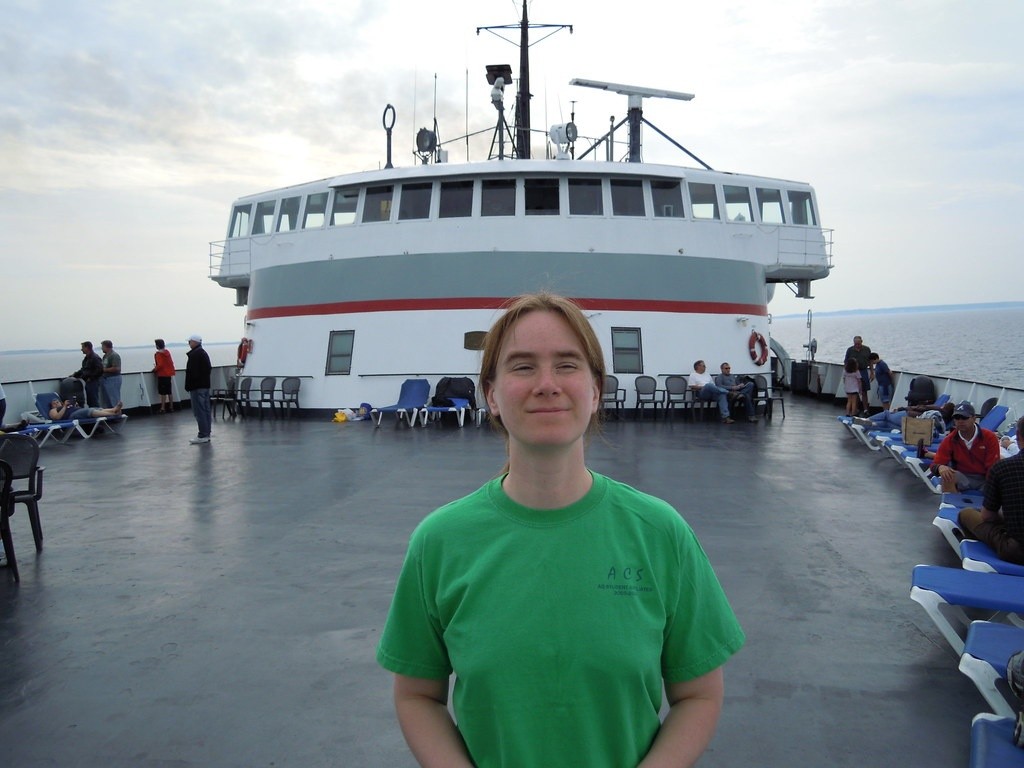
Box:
[210,377,299,422]
[370,377,486,430]
[598,374,786,426]
[832,395,1024,768]
[0,378,128,582]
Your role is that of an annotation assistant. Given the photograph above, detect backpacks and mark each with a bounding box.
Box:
[358,403,372,419]
[906,378,936,407]
[740,376,759,398]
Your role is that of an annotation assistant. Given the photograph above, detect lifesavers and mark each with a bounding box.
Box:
[749,332,768,365]
[238,338,248,367]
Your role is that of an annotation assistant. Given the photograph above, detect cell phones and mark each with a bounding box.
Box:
[963,499,972,503]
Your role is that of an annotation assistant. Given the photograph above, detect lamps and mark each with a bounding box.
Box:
[550,122,577,160]
[416,127,437,164]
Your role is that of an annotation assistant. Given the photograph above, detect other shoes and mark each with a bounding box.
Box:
[189,437,210,443]
[748,416,759,422]
[1007,650,1024,747]
[727,392,739,403]
[737,394,745,400]
[720,417,735,424]
[850,416,872,430]
[863,409,870,415]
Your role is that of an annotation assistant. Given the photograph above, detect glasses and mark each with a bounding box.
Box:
[954,415,970,420]
[725,367,730,369]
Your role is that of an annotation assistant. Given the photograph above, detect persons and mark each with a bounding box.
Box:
[376,292,747,766]
[916,404,1024,494]
[151,339,176,414]
[842,335,895,418]
[957,415,1024,566]
[48,340,123,424]
[0,381,29,435]
[688,360,760,424]
[185,335,212,443]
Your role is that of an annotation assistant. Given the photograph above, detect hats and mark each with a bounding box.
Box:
[185,335,203,343]
[951,404,974,418]
